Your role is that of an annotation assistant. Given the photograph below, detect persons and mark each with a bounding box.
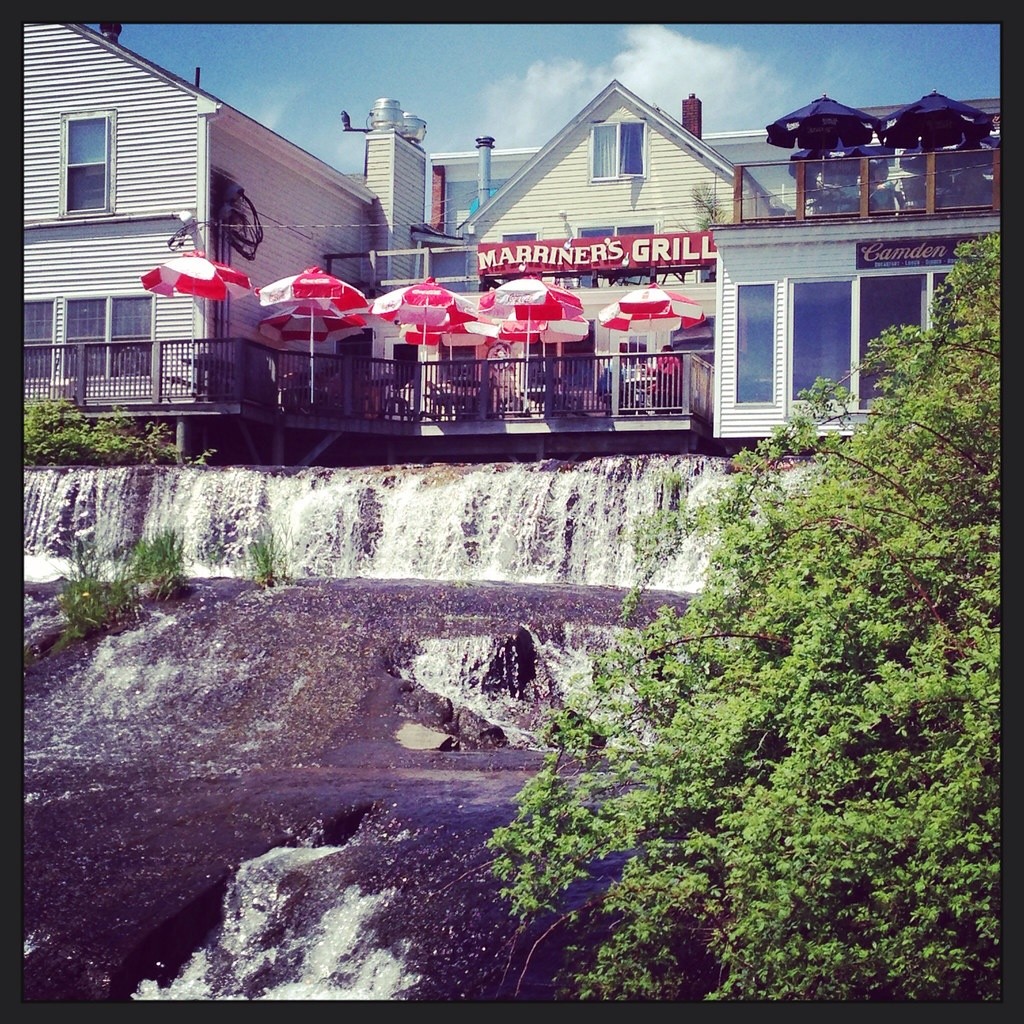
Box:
[813,169,840,213]
[869,175,895,206]
[597,359,636,415]
[653,345,683,414]
[599,362,634,415]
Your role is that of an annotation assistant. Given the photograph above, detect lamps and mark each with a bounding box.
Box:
[559,210,568,223]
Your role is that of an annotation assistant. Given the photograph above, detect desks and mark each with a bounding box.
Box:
[519,387,561,403]
[183,359,230,402]
[447,380,489,396]
[624,377,657,387]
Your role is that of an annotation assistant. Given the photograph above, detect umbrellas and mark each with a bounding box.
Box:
[597,282,706,415]
[254,266,590,409]
[140,253,252,395]
[766,89,1001,215]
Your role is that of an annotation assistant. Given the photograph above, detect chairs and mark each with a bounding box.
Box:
[426,381,460,421]
[597,369,682,416]
